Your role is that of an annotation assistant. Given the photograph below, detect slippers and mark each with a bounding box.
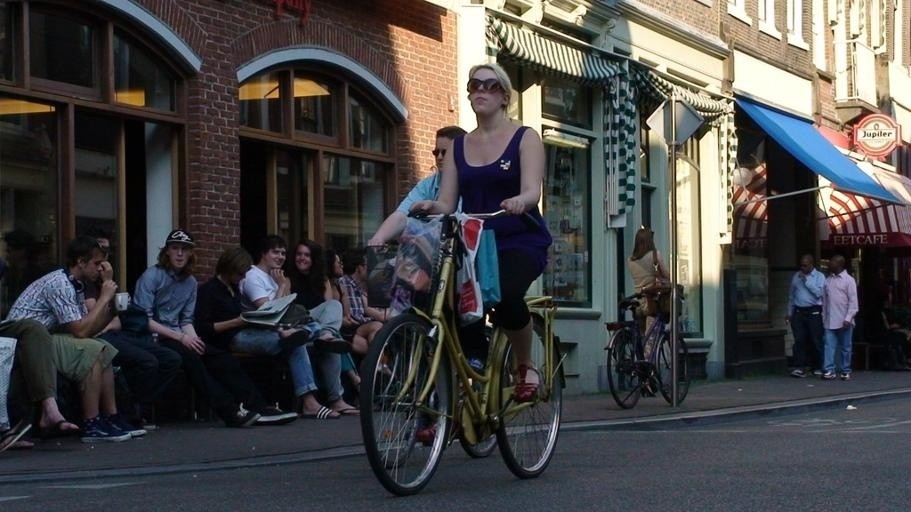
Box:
[278,329,353,354]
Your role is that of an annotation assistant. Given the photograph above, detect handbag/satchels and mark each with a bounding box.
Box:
[388,213,501,331]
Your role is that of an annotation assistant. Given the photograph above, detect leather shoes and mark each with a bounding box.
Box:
[416,422,437,442]
[512,363,539,403]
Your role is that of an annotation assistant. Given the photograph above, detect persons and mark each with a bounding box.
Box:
[366,124,468,441]
[403,61,553,445]
[134,229,400,429]
[626,227,669,361]
[784,254,825,378]
[820,254,859,380]
[1,227,183,453]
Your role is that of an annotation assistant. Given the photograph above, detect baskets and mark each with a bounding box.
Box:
[367,246,397,307]
[646,284,683,315]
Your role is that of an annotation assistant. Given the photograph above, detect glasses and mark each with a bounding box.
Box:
[467,78,503,93]
[432,149,446,156]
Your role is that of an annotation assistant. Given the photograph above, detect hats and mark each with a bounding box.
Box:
[166,229,198,248]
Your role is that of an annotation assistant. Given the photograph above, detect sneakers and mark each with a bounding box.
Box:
[234,402,360,428]
[0,412,160,454]
[790,368,850,380]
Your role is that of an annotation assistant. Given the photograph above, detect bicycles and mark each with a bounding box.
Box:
[602,278,693,410]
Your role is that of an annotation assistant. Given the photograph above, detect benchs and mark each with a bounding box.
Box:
[153,352,265,421]
[853,340,887,371]
